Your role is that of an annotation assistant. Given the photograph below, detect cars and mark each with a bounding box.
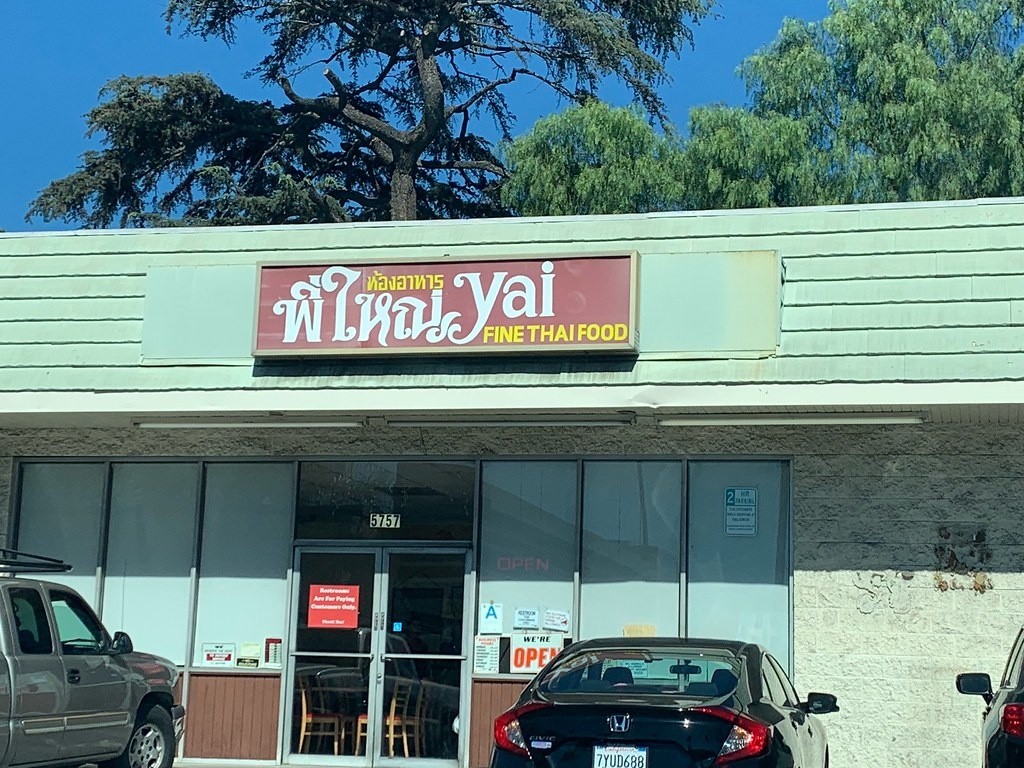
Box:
[489,636,839,768]
[954,622,1024,768]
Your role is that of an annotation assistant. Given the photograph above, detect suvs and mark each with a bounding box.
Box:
[0,547,185,768]
[295,622,460,750]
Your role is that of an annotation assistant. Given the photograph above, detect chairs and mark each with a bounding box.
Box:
[299,673,431,757]
[19,630,34,644]
[582,680,611,690]
[688,682,718,695]
[712,669,738,693]
[604,667,633,685]
